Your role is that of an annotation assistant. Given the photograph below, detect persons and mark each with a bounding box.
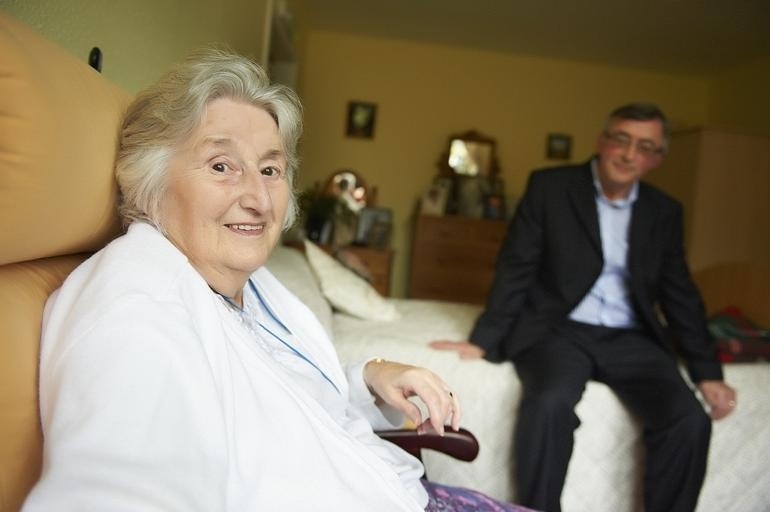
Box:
[16,40,533,511]
[427,100,739,511]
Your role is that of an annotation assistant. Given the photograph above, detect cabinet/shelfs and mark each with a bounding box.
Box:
[641,125,768,326]
[411,215,515,301]
[287,242,394,295]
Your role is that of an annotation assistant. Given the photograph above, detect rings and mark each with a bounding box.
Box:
[448,392,454,398]
[729,399,737,407]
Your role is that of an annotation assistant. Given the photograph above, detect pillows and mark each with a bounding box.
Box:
[300,235,398,322]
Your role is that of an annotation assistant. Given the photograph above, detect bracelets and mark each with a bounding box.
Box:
[373,357,390,364]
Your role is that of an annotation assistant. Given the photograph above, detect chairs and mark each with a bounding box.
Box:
[1,13,479,512]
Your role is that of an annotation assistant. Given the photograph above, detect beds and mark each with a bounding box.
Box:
[270,243,769,510]
